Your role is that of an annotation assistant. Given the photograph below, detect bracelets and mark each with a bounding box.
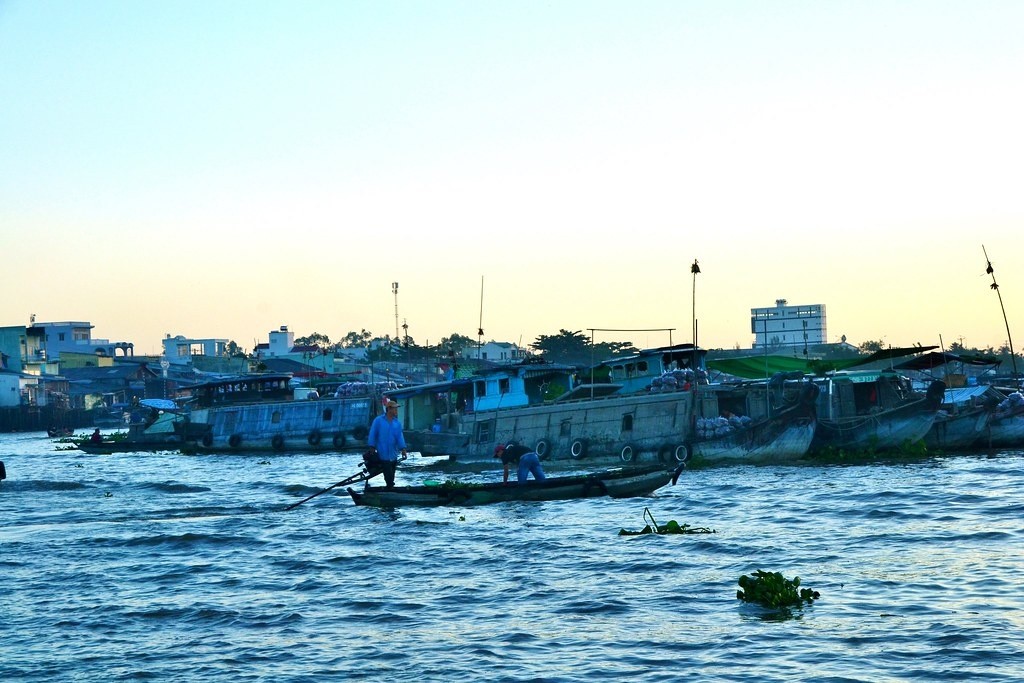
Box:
[402,448,406,451]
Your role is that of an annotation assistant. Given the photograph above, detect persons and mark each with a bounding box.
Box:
[496,444,545,484]
[91,429,101,440]
[368,401,407,492]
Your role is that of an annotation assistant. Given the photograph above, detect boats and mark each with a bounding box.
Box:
[347,447,685,509]
[47,429,74,437]
[78,318,1024,474]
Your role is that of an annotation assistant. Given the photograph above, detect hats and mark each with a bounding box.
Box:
[386,401,401,408]
[493,444,504,458]
